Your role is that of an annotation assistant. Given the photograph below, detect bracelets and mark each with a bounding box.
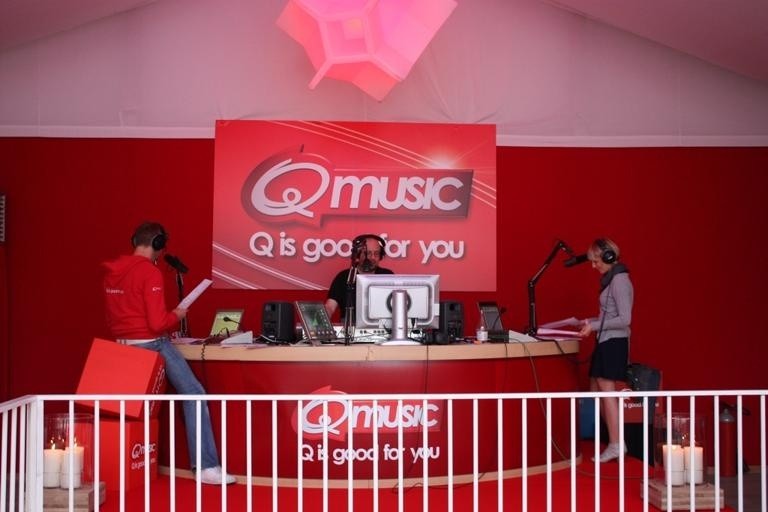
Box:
[584,318,588,325]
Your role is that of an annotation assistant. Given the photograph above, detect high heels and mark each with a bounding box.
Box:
[591,440,628,465]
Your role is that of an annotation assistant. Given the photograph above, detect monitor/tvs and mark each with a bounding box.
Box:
[355,275,439,347]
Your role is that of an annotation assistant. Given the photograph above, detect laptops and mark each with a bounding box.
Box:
[207,309,243,343]
[479,298,516,343]
[295,300,344,346]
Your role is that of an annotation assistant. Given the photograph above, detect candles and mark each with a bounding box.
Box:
[43,437,84,489]
[662,444,704,486]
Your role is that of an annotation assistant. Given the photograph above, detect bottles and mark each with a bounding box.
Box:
[477,309,488,341]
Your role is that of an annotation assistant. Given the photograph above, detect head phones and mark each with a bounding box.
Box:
[131,219,165,252]
[595,239,615,263]
[351,233,387,260]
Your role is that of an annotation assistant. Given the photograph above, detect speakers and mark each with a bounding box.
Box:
[439,300,466,345]
[260,301,294,343]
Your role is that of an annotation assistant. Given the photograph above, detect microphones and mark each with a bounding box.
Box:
[219,314,250,342]
[565,248,588,268]
[163,255,194,275]
[359,257,374,277]
[493,305,508,332]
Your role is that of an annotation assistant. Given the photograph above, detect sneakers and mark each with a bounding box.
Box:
[195,462,237,485]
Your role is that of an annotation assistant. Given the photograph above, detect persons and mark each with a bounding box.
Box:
[577,235,634,464]
[102,222,236,485]
[323,234,395,326]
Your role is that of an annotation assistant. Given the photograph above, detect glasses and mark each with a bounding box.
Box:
[359,250,382,258]
